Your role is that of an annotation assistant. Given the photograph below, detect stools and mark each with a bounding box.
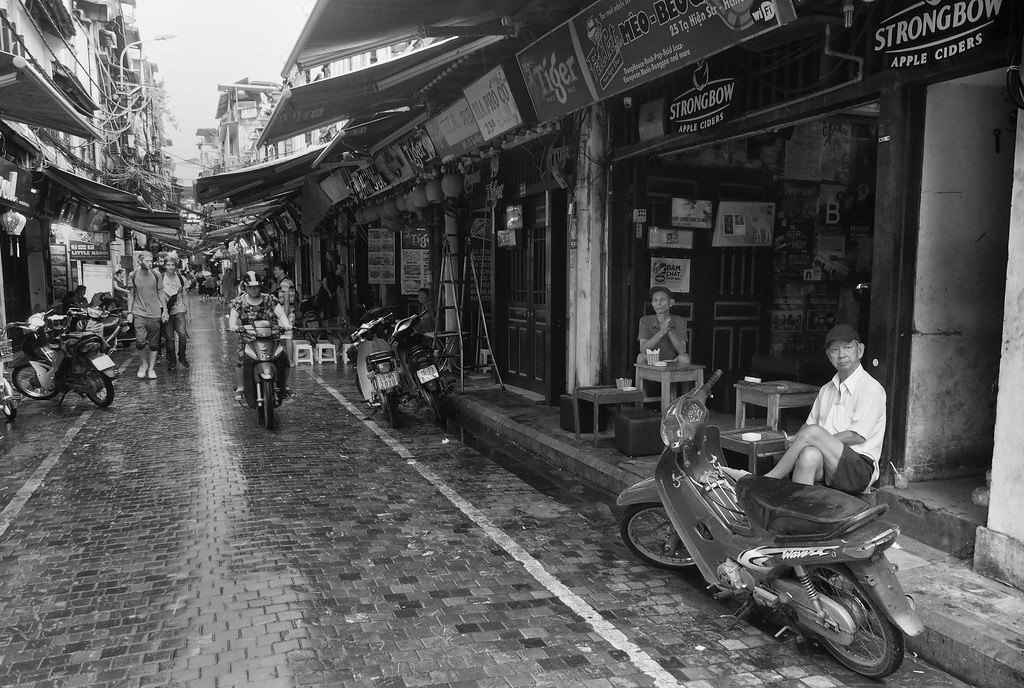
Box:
[557,394,608,434]
[617,411,665,455]
[342,344,354,364]
[479,349,495,373]
[294,344,314,367]
[316,343,338,365]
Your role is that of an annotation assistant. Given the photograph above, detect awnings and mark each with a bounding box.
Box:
[255,0,547,150]
[0,50,424,254]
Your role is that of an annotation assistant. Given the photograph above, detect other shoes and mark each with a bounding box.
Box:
[180,357,189,367]
[156,360,162,363]
[148,369,157,378]
[234,390,245,400]
[168,359,177,370]
[137,361,148,378]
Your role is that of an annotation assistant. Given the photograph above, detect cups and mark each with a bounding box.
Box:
[646,354,659,365]
[615,378,632,391]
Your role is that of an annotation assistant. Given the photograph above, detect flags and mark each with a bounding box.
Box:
[301,172,333,236]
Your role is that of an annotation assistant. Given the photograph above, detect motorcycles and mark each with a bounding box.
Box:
[4,308,120,408]
[390,308,457,423]
[48,303,136,356]
[617,369,925,679]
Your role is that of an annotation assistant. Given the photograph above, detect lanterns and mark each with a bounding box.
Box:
[354,173,463,225]
[0,210,19,256]
[13,212,27,257]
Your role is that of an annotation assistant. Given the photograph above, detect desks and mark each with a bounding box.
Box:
[425,330,469,375]
[732,380,823,430]
[716,428,795,474]
[635,362,705,413]
[298,327,348,348]
[571,384,642,449]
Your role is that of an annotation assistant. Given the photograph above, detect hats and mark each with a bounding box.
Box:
[824,323,861,348]
[649,286,673,300]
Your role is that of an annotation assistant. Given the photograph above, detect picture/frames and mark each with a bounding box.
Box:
[771,310,803,332]
[807,310,838,331]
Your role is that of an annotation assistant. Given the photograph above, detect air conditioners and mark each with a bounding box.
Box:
[107,134,122,157]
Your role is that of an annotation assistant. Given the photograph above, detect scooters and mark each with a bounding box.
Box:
[225,320,298,429]
[347,312,410,428]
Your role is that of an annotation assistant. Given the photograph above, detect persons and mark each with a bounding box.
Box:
[228,271,297,401]
[418,287,443,318]
[273,262,296,367]
[113,269,130,312]
[184,267,235,318]
[774,314,801,329]
[316,264,347,319]
[262,268,270,282]
[635,286,690,394]
[725,216,732,232]
[126,250,169,378]
[159,256,192,370]
[765,324,887,496]
[62,285,89,315]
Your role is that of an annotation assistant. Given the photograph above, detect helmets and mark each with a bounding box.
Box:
[243,271,262,287]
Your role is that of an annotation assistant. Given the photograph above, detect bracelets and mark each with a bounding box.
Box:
[128,312,134,315]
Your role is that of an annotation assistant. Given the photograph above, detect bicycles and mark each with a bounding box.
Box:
[0,322,32,419]
